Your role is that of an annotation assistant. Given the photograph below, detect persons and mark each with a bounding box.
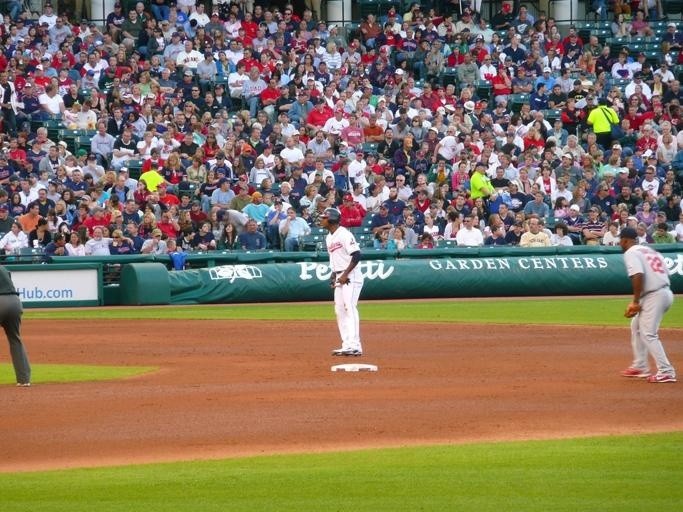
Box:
[319,208,364,356]
[0,265,31,387]
[1,1,683,254]
[619,227,677,383]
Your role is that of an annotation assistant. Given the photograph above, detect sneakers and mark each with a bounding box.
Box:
[332,349,362,356]
[620,367,677,383]
[16,383,31,387]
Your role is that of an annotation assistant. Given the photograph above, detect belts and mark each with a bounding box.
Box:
[640,284,669,299]
[337,271,342,274]
[0,292,18,295]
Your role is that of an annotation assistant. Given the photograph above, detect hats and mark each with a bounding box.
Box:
[0,0,682,237]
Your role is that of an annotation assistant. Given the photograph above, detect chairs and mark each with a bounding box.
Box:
[32,20,683,251]
